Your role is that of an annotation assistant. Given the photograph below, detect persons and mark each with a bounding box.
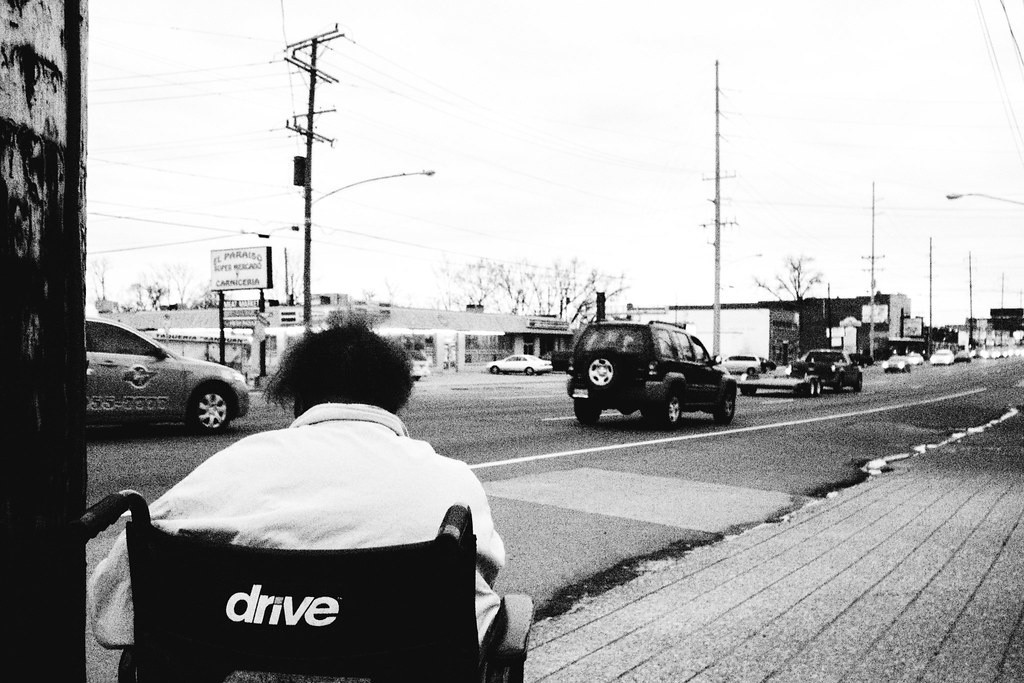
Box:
[87,320,504,649]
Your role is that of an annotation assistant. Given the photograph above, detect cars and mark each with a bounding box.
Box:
[928,350,955,366]
[541,350,574,374]
[882,355,911,374]
[84,312,250,437]
[719,354,769,377]
[970,347,1023,360]
[485,353,554,378]
[801,348,863,394]
[953,351,972,363]
[905,352,924,366]
[405,350,430,381]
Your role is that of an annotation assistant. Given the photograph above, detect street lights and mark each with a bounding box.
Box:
[302,170,437,333]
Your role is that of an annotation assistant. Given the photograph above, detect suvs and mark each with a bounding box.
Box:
[565,320,737,431]
